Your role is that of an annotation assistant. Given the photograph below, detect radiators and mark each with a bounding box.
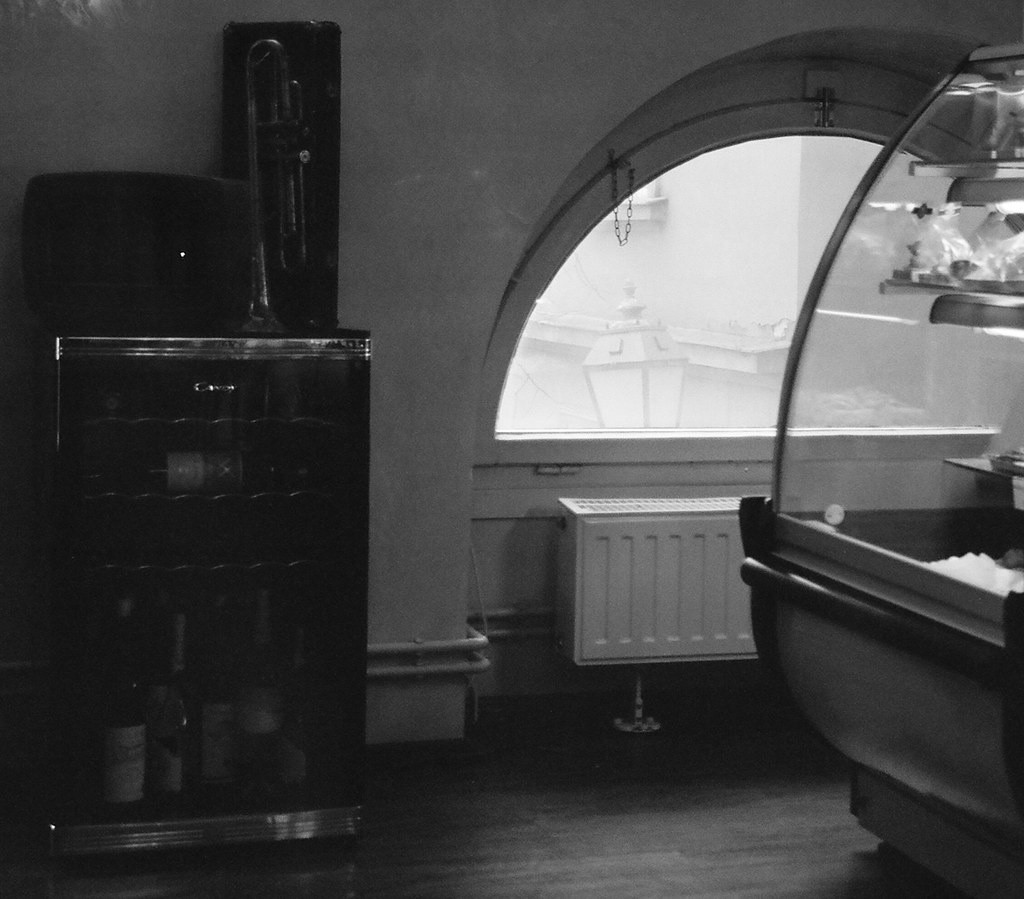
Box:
[554,495,759,666]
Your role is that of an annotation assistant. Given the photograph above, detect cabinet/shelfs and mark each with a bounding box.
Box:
[737,40,1024,899]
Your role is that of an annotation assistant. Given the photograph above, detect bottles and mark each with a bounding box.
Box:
[143,452,312,492]
[96,583,331,808]
